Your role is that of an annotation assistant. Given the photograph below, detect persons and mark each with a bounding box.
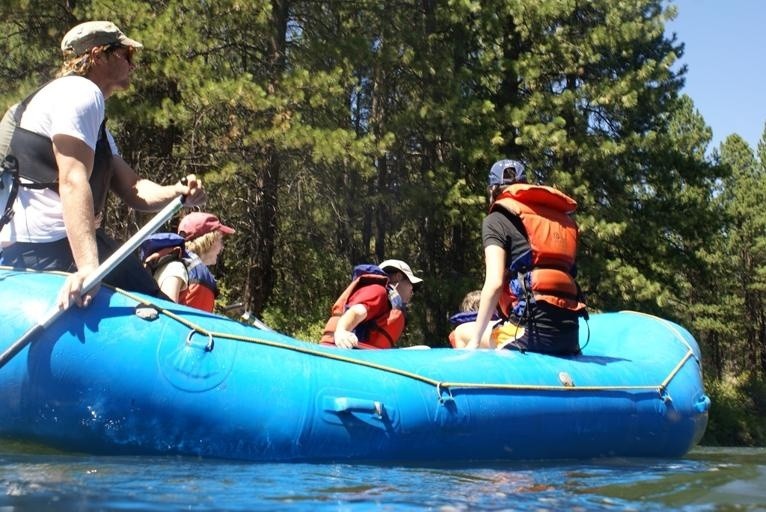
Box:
[448,289,482,348]
[0,18,207,309]
[144,210,236,313]
[317,258,431,349]
[448,157,581,355]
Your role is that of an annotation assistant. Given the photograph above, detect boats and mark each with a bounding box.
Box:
[0,265,712,467]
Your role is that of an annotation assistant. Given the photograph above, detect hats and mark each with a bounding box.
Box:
[62,21,144,58]
[178,212,233,245]
[378,258,423,285]
[490,159,526,184]
